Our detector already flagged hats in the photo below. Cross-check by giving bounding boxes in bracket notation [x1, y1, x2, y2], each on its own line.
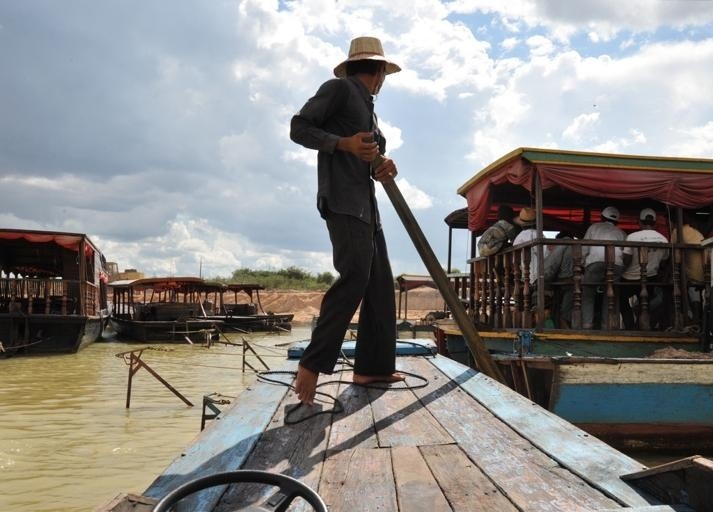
[511, 207, 538, 228]
[637, 207, 657, 224]
[599, 205, 620, 222]
[334, 37, 402, 79]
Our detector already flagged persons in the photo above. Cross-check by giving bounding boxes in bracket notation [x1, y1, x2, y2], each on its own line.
[288, 36, 408, 407]
[477, 204, 713, 331]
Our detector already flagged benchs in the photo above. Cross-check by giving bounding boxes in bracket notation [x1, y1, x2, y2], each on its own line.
[478, 282, 708, 331]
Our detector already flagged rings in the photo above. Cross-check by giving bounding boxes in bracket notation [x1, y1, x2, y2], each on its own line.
[388, 172, 392, 176]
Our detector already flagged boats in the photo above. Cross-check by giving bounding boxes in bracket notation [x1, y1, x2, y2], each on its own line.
[429, 146, 712, 369]
[311, 272, 438, 342]
[0, 229, 295, 355]
[489, 351, 713, 452]
